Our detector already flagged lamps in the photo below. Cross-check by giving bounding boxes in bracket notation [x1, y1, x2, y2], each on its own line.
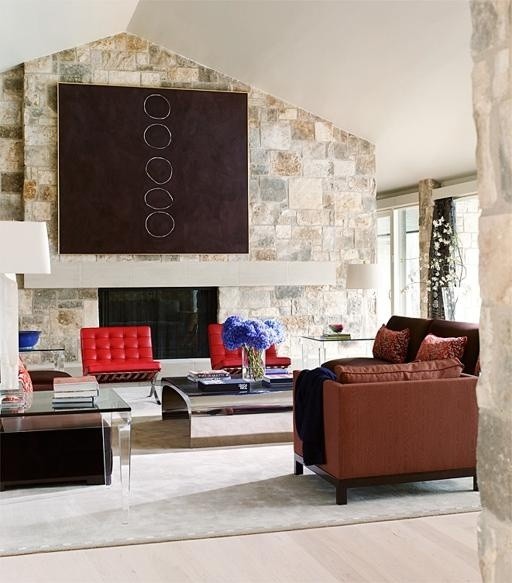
[0, 221, 51, 276]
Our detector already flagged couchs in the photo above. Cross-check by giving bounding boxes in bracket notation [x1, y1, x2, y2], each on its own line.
[291, 316, 480, 506]
[80, 325, 160, 383]
[208, 323, 291, 374]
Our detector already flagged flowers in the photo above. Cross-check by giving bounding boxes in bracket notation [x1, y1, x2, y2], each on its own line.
[222, 315, 286, 351]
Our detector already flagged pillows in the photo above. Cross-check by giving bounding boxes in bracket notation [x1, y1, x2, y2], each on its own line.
[416, 332, 468, 366]
[373, 323, 411, 364]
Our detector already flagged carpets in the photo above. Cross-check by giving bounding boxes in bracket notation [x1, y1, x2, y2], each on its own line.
[1, 381, 480, 557]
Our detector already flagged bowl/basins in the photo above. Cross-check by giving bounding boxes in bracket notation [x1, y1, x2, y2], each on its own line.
[19, 329, 42, 348]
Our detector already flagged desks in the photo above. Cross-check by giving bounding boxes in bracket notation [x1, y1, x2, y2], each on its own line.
[0, 371, 133, 490]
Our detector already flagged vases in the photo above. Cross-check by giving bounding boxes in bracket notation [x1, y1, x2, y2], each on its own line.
[241, 348, 267, 382]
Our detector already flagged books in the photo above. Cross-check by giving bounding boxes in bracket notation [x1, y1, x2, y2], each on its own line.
[186, 369, 231, 383]
[51, 374, 100, 410]
[262, 373, 294, 390]
